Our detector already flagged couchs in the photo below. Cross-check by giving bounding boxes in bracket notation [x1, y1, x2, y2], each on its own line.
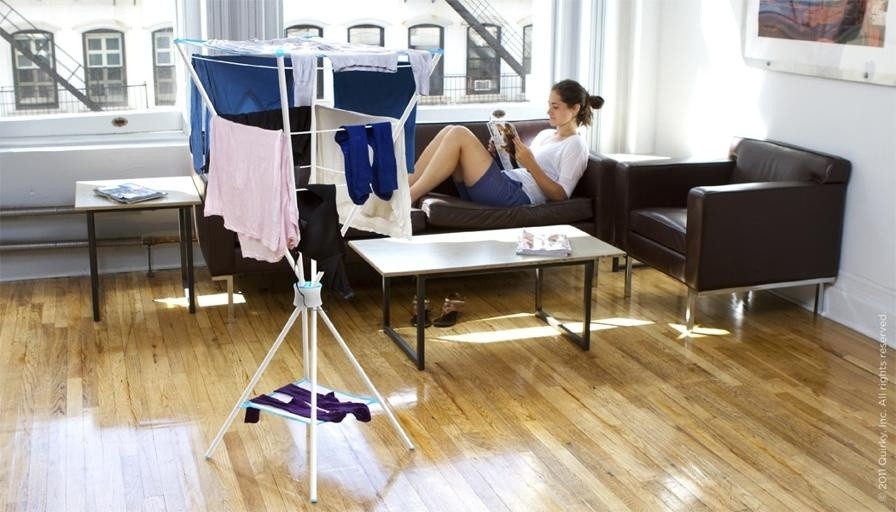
[194, 120, 617, 293]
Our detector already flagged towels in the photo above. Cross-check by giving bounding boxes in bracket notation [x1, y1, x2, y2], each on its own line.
[184, 51, 420, 264]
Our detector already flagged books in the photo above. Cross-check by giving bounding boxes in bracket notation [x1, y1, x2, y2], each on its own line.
[93, 183, 167, 204]
[485, 109, 520, 169]
[517, 229, 572, 258]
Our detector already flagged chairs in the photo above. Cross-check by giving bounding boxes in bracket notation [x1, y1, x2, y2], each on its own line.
[619, 138, 852, 332]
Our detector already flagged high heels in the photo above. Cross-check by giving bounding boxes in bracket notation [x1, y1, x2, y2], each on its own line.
[411, 292, 464, 327]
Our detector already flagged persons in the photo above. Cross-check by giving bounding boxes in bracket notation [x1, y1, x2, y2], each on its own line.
[408, 79, 606, 207]
